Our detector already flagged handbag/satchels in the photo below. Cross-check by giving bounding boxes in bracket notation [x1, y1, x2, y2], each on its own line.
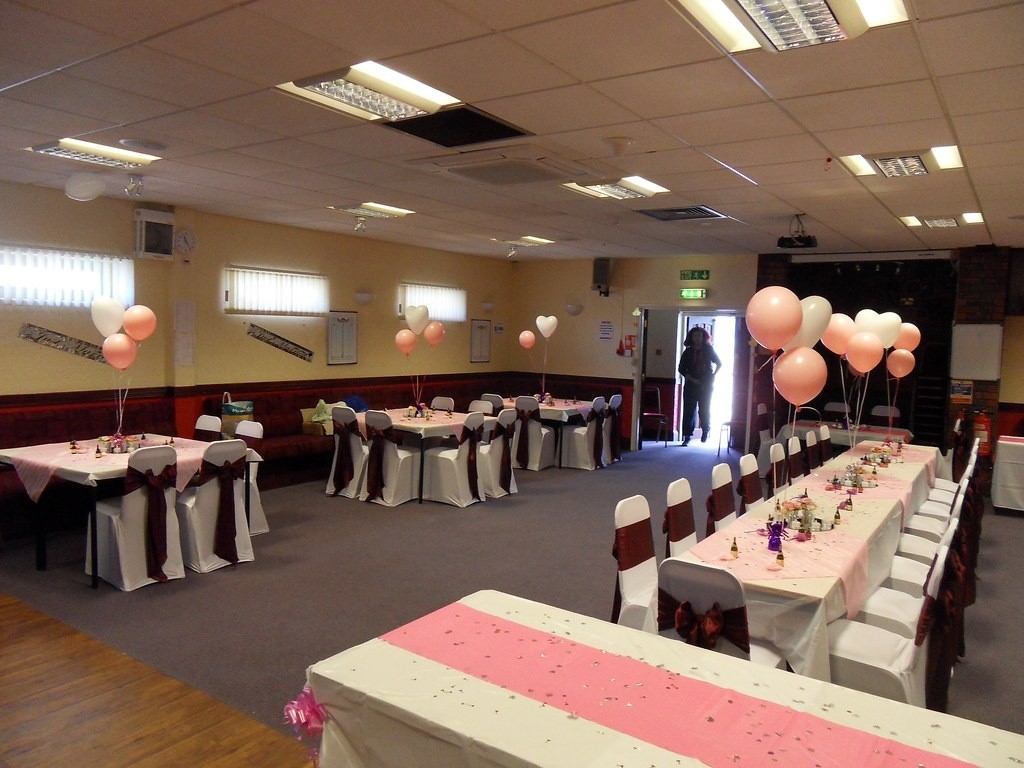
[222, 392, 254, 415]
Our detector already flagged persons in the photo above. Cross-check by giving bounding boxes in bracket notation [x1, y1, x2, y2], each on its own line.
[678, 327, 722, 445]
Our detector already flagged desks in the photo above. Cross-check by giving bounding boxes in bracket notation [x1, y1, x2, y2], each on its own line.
[357, 405, 497, 505]
[501, 396, 606, 466]
[675, 439, 953, 686]
[778, 420, 913, 456]
[306, 588, 1024, 768]
[0, 433, 263, 588]
[992, 436, 1024, 512]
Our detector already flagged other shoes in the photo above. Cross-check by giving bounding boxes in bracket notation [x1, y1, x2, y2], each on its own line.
[682, 436, 690, 446]
[701, 431, 708, 442]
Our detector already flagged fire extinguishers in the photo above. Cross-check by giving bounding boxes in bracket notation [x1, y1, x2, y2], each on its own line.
[973, 408, 992, 456]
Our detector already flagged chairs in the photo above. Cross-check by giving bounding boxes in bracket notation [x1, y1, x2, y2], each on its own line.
[84, 387, 985, 705]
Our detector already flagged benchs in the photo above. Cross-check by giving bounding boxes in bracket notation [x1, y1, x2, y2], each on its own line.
[1, 378, 634, 505]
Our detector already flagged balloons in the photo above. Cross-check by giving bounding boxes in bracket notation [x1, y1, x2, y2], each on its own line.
[102, 333, 138, 372]
[122, 305, 158, 341]
[855, 309, 879, 332]
[405, 305, 428, 336]
[887, 349, 915, 380]
[893, 323, 921, 352]
[536, 316, 558, 338]
[394, 329, 417, 356]
[745, 286, 803, 355]
[879, 312, 902, 349]
[821, 313, 856, 358]
[772, 347, 828, 412]
[519, 330, 536, 349]
[91, 296, 125, 337]
[782, 296, 832, 352]
[423, 321, 447, 349]
[846, 332, 884, 377]
